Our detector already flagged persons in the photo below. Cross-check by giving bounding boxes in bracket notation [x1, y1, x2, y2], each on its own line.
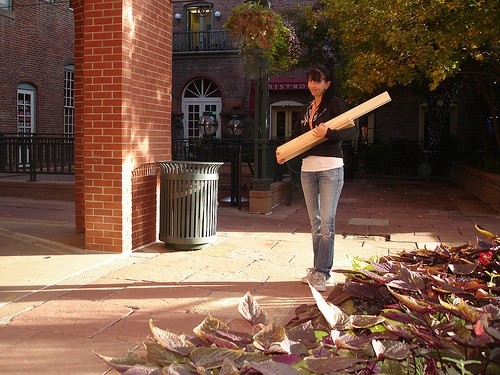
[276, 66, 360, 293]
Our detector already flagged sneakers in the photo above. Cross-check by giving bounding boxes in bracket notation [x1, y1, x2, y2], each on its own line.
[302, 267, 315, 283]
[311, 271, 327, 291]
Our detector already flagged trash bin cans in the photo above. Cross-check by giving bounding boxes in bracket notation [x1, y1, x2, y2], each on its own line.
[156, 161, 224, 250]
